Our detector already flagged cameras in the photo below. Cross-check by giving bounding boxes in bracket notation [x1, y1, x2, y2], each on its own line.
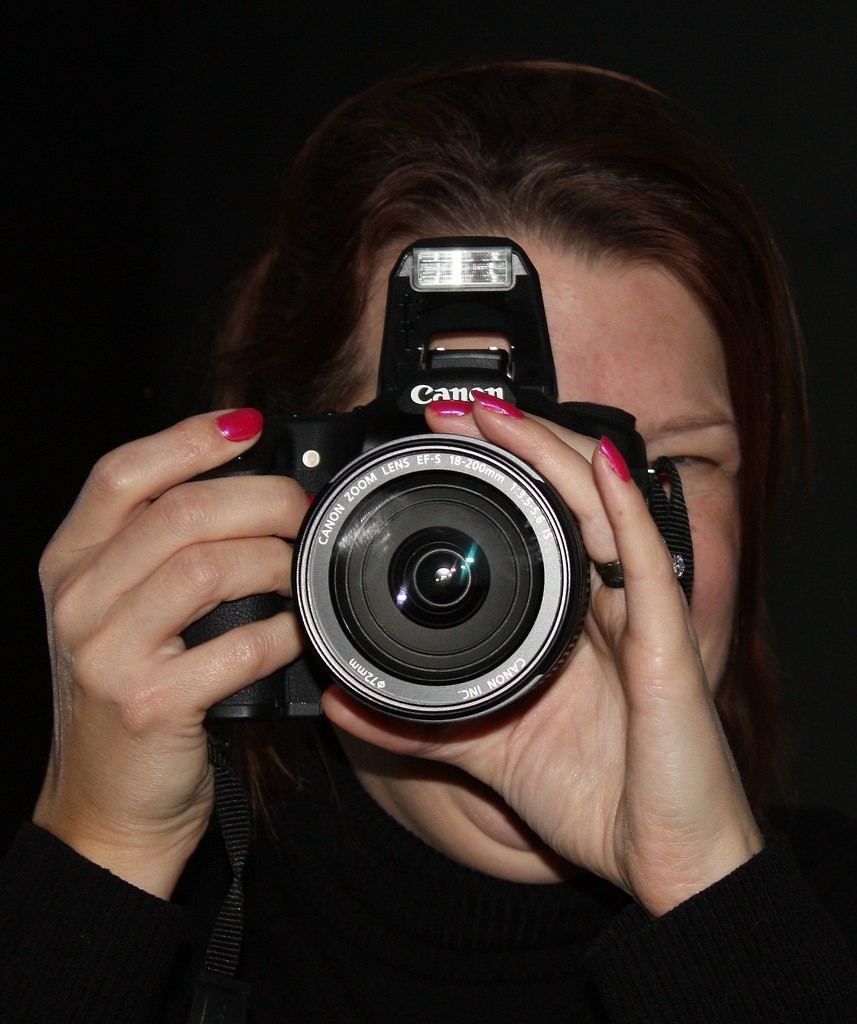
[178, 235, 650, 723]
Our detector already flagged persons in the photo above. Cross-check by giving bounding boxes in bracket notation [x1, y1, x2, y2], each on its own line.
[0, 60, 857, 1024]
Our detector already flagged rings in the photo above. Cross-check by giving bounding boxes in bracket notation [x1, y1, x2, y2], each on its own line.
[594, 554, 686, 588]
